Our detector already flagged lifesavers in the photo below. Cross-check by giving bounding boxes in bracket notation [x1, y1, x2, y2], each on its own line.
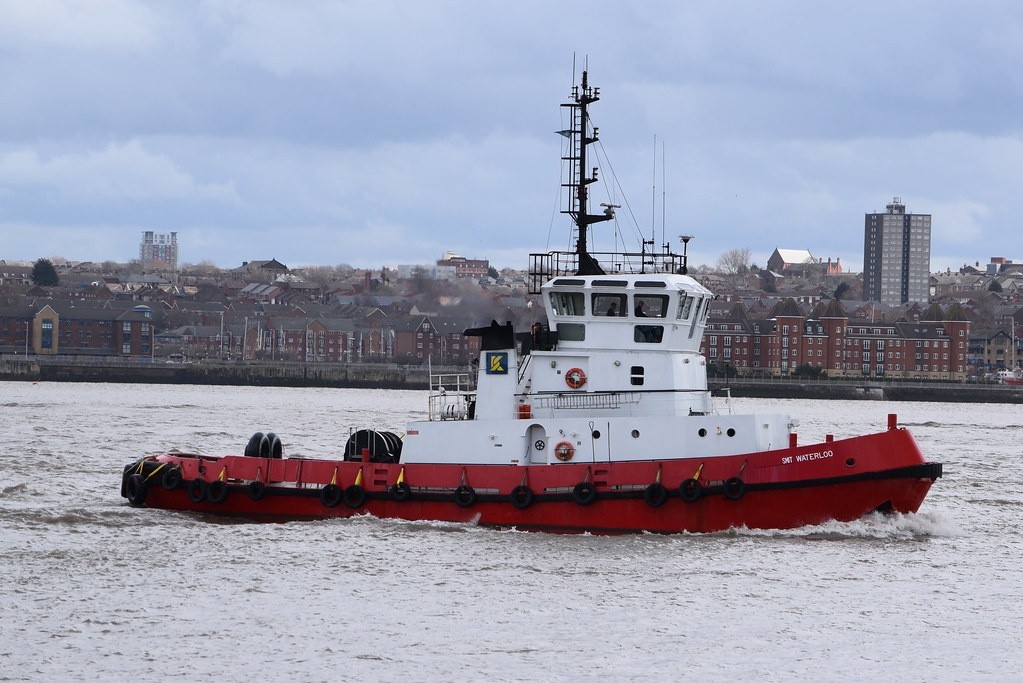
[644, 483, 666, 507]
[162, 469, 182, 491]
[320, 484, 342, 507]
[566, 368, 585, 389]
[206, 481, 227, 502]
[510, 485, 533, 508]
[121, 464, 134, 498]
[679, 478, 703, 502]
[723, 476, 744, 500]
[573, 482, 595, 504]
[125, 474, 146, 506]
[391, 482, 411, 502]
[342, 485, 366, 508]
[247, 481, 265, 500]
[555, 441, 574, 461]
[188, 478, 206, 502]
[454, 485, 475, 507]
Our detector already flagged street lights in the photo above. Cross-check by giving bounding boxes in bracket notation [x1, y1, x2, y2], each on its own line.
[1007, 315, 1016, 370]
[219, 314, 224, 361]
[24, 321, 28, 361]
[243, 315, 247, 362]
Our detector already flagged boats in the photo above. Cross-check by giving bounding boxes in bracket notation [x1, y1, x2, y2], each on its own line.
[118, 49, 944, 524]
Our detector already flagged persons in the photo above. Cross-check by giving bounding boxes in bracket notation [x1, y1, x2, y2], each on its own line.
[607, 303, 617, 316]
[635, 301, 647, 316]
